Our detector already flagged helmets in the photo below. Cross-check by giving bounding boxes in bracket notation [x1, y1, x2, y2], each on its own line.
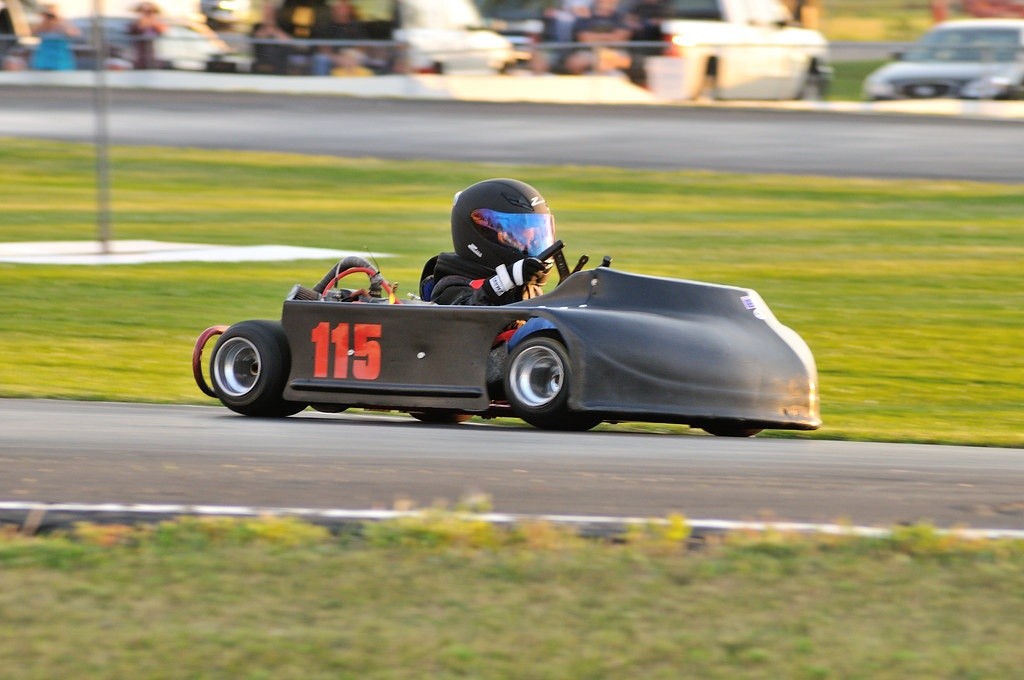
[451, 178, 556, 270]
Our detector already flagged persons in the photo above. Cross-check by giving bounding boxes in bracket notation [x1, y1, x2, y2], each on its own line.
[529, 0, 666, 77]
[0, 0, 405, 83]
[432, 178, 556, 344]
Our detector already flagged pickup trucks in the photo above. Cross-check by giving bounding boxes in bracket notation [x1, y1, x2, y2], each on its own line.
[643, 0, 833, 101]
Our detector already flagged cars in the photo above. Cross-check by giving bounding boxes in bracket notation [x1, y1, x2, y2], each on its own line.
[861, 19, 1024, 101]
[356, 0, 514, 75]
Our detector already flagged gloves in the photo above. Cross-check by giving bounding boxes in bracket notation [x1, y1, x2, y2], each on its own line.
[485, 257, 547, 307]
